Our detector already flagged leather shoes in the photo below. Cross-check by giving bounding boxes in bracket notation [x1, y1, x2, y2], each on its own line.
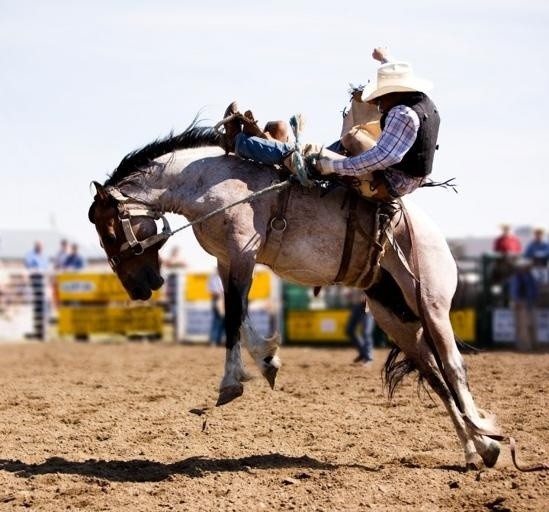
[219, 101, 241, 153]
[243, 110, 263, 137]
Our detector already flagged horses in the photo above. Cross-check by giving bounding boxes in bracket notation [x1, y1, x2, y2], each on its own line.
[86, 114, 502, 474]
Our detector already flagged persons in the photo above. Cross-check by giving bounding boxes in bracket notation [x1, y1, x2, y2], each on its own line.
[220, 44, 442, 204]
[507, 257, 541, 351]
[64, 243, 84, 267]
[495, 225, 523, 256]
[55, 239, 71, 264]
[165, 246, 188, 267]
[24, 240, 53, 268]
[343, 288, 375, 366]
[206, 268, 224, 345]
[525, 228, 549, 285]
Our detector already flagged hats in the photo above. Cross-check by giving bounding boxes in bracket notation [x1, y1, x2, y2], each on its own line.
[361, 62, 433, 103]
